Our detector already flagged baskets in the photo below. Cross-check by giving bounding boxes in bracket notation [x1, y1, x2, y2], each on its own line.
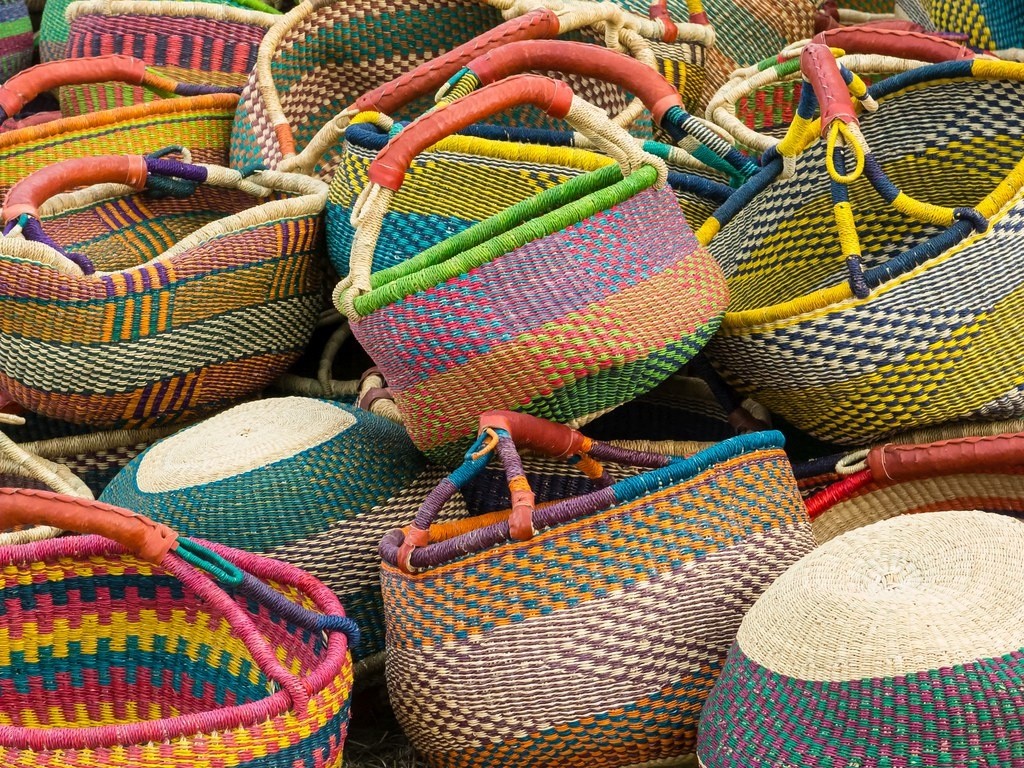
[0, 0, 1024, 768]
